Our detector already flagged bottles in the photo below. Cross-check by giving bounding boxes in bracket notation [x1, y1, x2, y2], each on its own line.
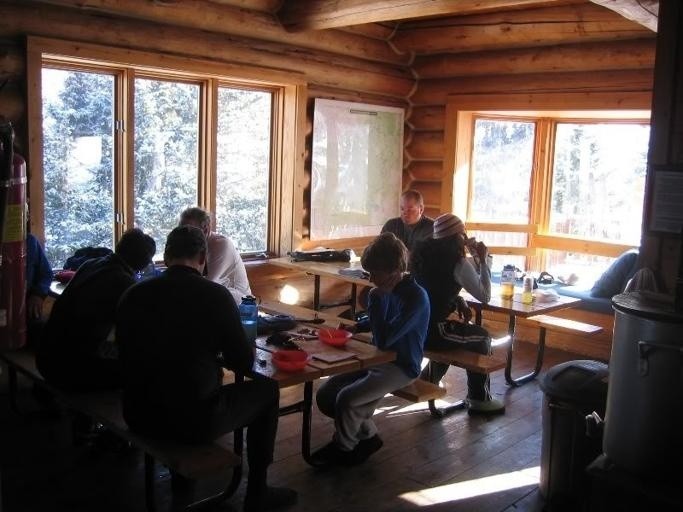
[522, 271, 533, 304]
[500, 263, 515, 300]
[237, 293, 259, 346]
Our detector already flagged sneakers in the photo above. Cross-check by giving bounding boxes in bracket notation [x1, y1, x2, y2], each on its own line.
[310, 433, 384, 472]
[469, 397, 506, 416]
[244, 486, 298, 512]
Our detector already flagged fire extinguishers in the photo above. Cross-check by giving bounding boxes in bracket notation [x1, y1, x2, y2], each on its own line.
[0, 115, 27, 353]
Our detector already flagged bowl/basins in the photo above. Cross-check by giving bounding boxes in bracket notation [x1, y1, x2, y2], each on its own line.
[270, 348, 313, 371]
[315, 327, 352, 346]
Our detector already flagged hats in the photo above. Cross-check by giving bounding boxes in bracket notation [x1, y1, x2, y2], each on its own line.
[433, 213, 465, 240]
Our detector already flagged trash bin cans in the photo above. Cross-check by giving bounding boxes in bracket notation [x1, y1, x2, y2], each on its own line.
[539, 360, 609, 506]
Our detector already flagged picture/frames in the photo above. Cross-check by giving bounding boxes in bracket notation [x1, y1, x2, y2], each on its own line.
[643, 163, 683, 239]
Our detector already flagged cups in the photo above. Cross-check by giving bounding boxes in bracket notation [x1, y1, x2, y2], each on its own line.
[465, 237, 477, 256]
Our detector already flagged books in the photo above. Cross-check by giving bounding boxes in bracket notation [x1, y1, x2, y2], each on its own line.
[337, 264, 369, 279]
[310, 349, 358, 364]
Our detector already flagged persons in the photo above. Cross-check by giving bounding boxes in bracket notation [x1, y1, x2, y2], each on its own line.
[24, 204, 53, 352]
[175, 208, 251, 310]
[412, 213, 505, 412]
[377, 191, 439, 269]
[34, 228, 156, 396]
[119, 223, 298, 510]
[307, 232, 433, 470]
